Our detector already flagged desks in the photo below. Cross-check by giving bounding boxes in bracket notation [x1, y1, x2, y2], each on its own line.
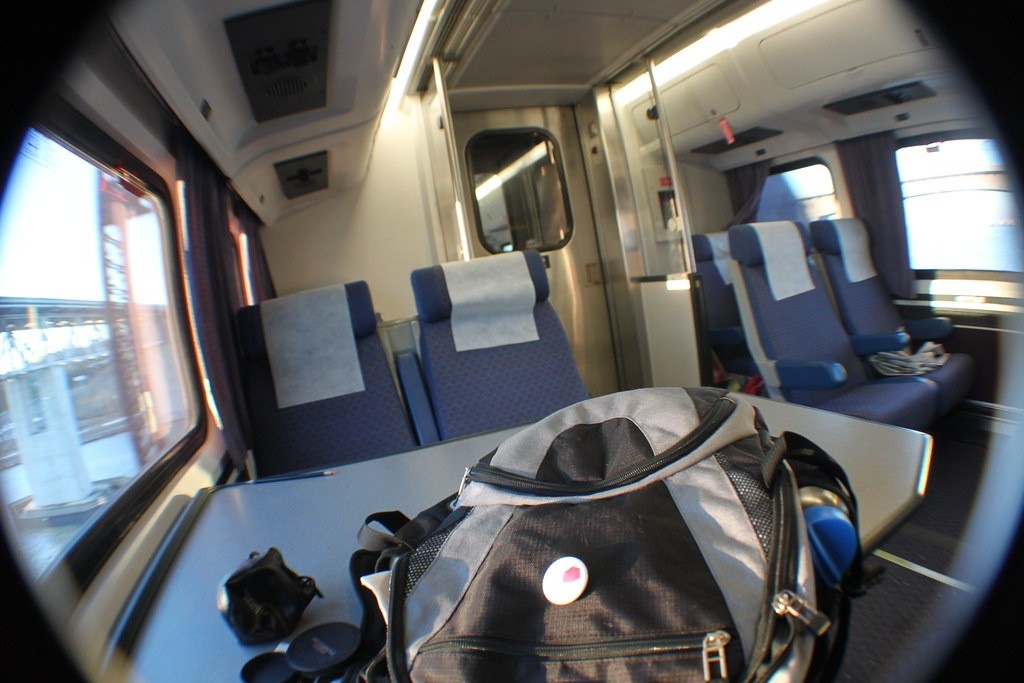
[117, 386, 936, 683]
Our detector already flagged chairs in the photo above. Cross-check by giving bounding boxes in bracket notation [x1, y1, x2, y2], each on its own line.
[409, 250, 591, 442]
[233, 280, 420, 469]
[810, 217, 974, 421]
[691, 230, 759, 379]
[728, 220, 943, 432]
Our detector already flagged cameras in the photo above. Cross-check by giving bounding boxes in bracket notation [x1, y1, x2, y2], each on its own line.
[216, 547, 324, 648]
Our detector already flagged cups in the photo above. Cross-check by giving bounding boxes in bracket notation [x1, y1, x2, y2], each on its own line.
[797, 486, 861, 589]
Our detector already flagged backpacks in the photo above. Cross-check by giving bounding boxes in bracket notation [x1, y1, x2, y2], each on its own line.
[313, 384, 886, 683]
